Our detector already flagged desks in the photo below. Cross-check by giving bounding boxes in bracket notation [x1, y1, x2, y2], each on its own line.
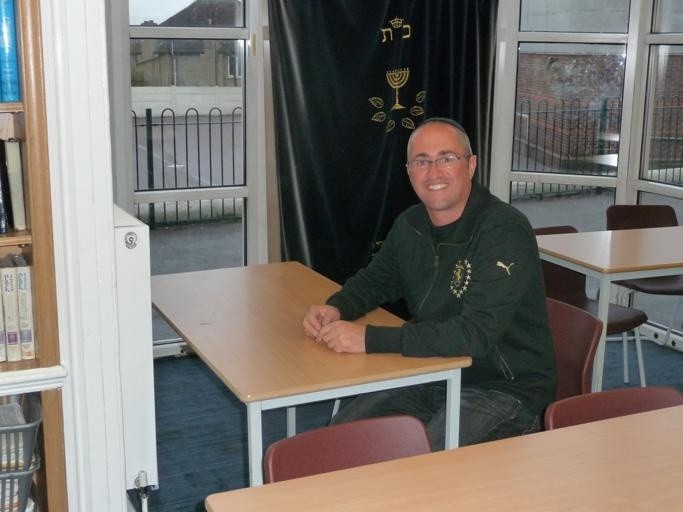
[529, 222, 681, 392]
[148, 260, 474, 490]
[201, 402, 682, 511]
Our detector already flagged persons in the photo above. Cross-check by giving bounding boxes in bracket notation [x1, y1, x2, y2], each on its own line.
[302, 117, 559, 453]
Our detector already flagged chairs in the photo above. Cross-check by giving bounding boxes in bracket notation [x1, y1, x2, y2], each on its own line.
[532, 225, 646, 389]
[543, 297, 603, 399]
[605, 201, 682, 387]
[260, 413, 430, 484]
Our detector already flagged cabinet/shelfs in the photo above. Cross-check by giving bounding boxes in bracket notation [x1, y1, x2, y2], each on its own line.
[0, 1, 72, 512]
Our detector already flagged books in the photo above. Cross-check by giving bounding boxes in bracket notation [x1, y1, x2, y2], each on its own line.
[1, 0, 37, 368]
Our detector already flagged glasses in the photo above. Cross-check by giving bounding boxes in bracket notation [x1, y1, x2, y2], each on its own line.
[405, 153, 471, 170]
